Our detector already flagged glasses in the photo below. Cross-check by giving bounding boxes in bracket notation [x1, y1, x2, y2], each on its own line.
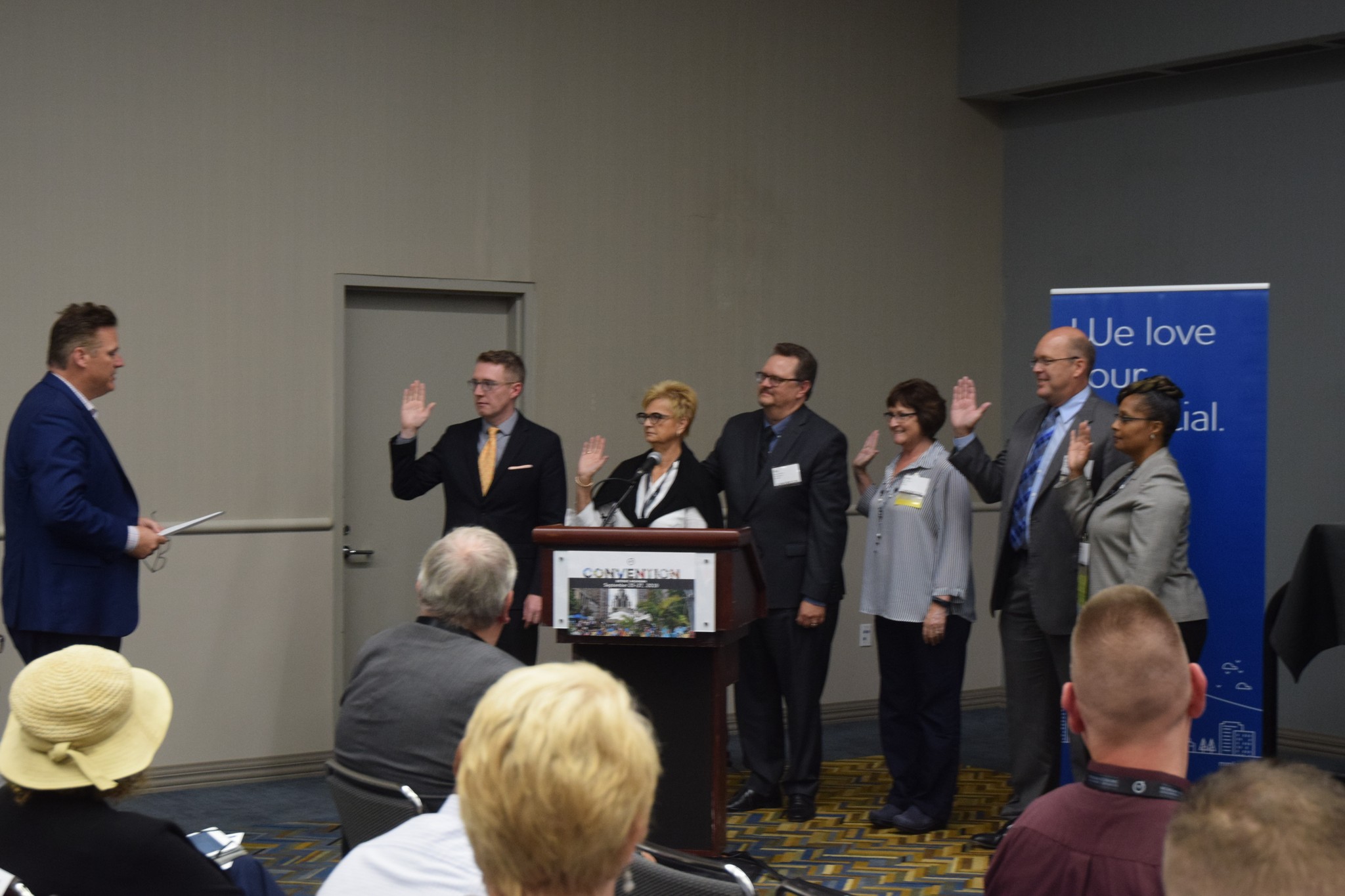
[466, 379, 513, 391]
[1113, 412, 1143, 423]
[755, 371, 800, 385]
[1029, 355, 1078, 365]
[883, 410, 913, 421]
[635, 412, 668, 424]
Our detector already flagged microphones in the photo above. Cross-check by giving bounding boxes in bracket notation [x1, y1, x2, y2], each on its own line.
[633, 452, 662, 481]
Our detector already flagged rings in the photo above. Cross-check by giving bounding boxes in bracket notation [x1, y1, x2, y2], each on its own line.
[587, 451, 592, 454]
[934, 632, 941, 634]
[810, 624, 818, 626]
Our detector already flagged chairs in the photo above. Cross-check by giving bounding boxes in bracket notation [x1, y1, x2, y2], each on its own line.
[615, 841, 857, 896]
[324, 759, 426, 862]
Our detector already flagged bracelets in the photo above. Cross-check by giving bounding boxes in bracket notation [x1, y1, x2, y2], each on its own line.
[575, 477, 594, 486]
[932, 597, 951, 607]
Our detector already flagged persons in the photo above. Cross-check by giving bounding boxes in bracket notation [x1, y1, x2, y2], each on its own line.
[1052, 376, 1211, 763]
[852, 378, 977, 833]
[333, 527, 527, 812]
[390, 352, 567, 667]
[312, 740, 487, 896]
[947, 325, 1132, 848]
[454, 662, 658, 896]
[1161, 759, 1345, 896]
[0, 645, 286, 896]
[700, 343, 851, 822]
[983, 583, 1207, 896]
[1, 302, 166, 665]
[573, 379, 723, 529]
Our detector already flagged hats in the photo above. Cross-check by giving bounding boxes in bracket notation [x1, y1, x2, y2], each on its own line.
[1, 644, 172, 791]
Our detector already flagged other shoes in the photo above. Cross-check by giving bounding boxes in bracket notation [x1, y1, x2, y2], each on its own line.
[868, 803, 901, 825]
[969, 820, 1016, 849]
[894, 806, 944, 832]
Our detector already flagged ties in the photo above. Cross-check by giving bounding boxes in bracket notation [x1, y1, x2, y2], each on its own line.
[759, 427, 771, 472]
[1009, 410, 1060, 551]
[478, 427, 499, 496]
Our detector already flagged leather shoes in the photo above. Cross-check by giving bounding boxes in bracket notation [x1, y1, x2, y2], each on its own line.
[726, 789, 782, 810]
[787, 793, 816, 821]
